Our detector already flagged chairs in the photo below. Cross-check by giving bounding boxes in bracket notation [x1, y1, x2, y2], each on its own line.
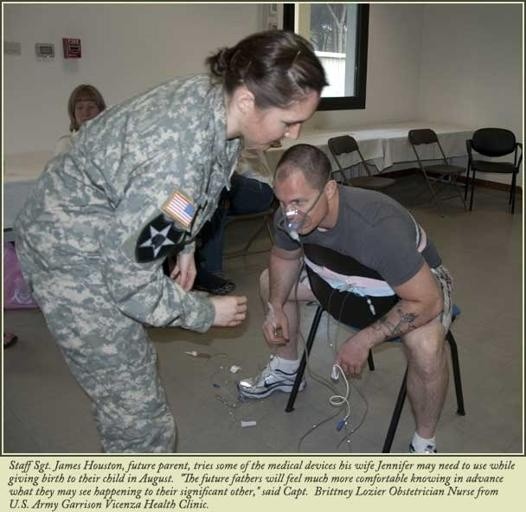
[408, 127, 522, 219]
[282, 243, 466, 454]
[327, 134, 397, 193]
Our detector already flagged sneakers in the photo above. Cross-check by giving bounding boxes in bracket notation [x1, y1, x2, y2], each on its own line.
[192, 276, 236, 296]
[236, 365, 304, 399]
[409, 442, 437, 453]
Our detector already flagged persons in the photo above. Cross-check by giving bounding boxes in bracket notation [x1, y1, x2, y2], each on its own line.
[234, 143, 454, 453]
[4, 331, 18, 348]
[66, 82, 239, 297]
[196, 139, 276, 277]
[14, 29, 331, 454]
